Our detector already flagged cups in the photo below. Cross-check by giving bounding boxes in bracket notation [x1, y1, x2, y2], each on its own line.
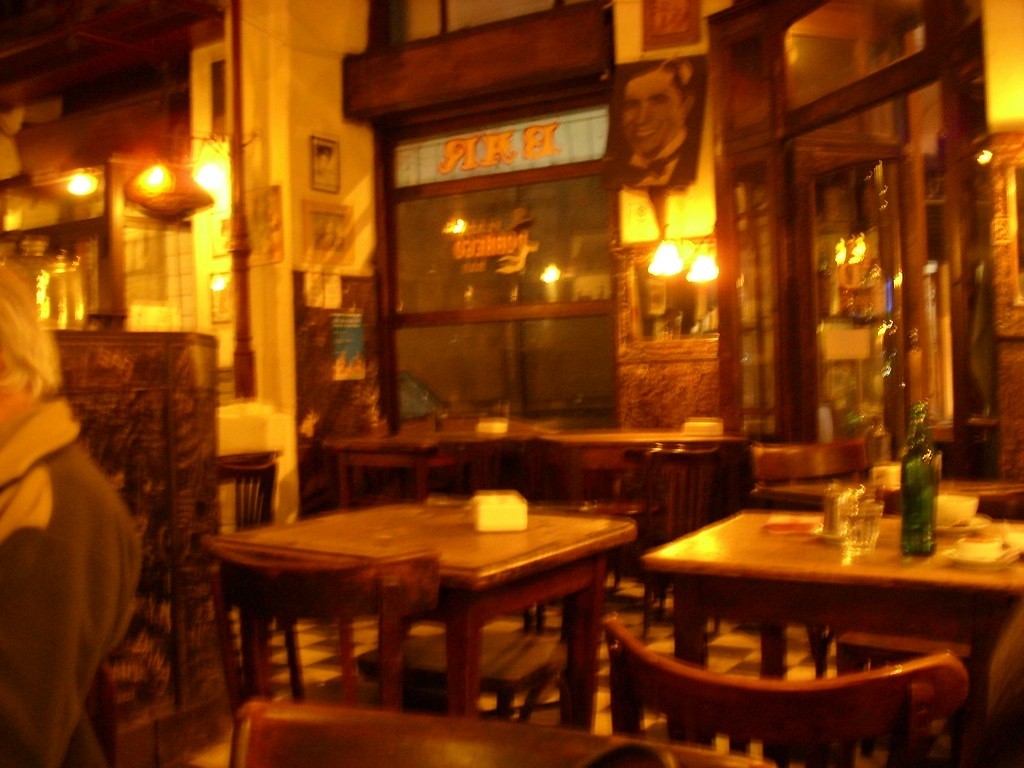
[936, 494, 979, 527]
[957, 533, 1003, 559]
[871, 463, 901, 492]
[820, 479, 884, 556]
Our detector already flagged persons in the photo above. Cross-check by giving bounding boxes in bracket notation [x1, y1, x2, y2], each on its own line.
[613, 59, 697, 184]
[0, 267, 139, 768]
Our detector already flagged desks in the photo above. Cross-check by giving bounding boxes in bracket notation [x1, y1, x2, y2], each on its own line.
[321, 435, 529, 515]
[642, 508, 1024, 767]
[529, 429, 749, 527]
[750, 470, 1024, 519]
[211, 497, 634, 733]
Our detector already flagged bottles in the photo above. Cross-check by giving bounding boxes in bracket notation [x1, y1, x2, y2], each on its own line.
[901, 398, 938, 554]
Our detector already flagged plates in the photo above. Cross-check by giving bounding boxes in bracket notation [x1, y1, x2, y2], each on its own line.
[943, 544, 1022, 571]
[935, 519, 990, 535]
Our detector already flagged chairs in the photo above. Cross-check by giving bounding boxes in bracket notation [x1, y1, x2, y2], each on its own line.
[202, 412, 1024, 768]
